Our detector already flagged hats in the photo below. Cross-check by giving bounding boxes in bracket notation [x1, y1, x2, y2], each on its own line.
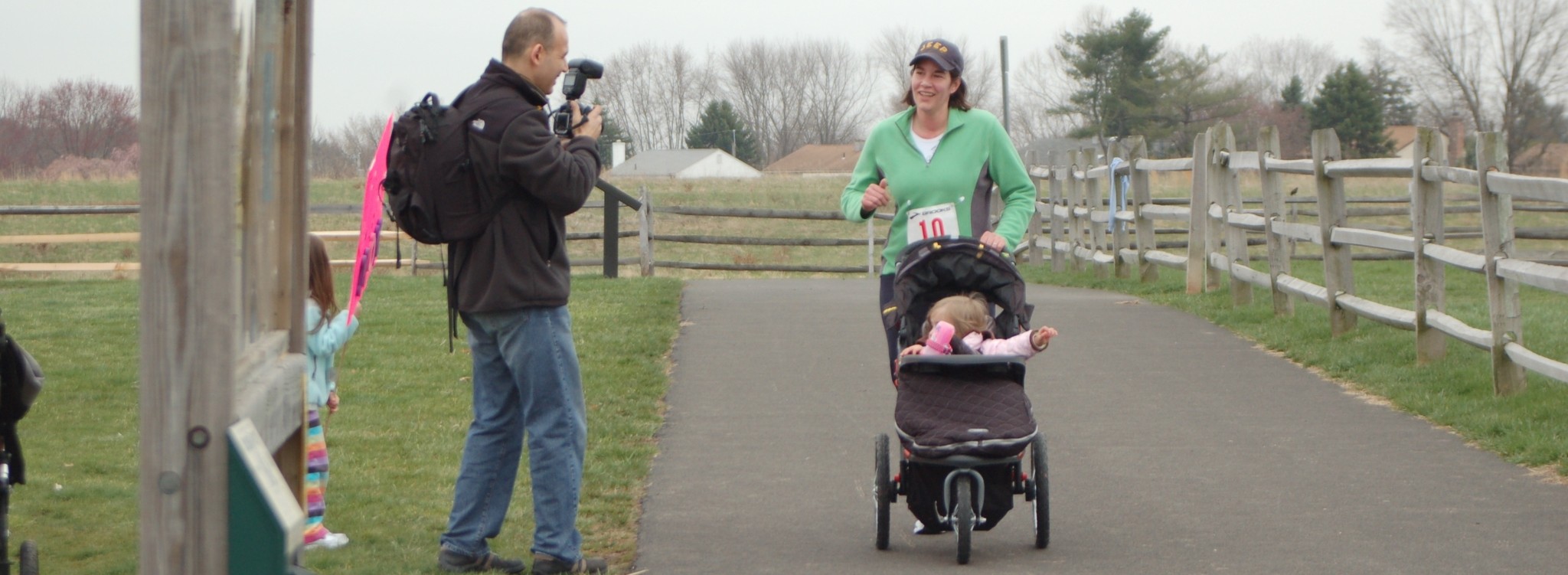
[910, 38, 965, 76]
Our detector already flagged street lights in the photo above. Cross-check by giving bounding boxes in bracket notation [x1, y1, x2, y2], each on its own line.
[732, 129, 736, 158]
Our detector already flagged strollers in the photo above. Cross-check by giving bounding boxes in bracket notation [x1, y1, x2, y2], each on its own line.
[871, 234, 1052, 565]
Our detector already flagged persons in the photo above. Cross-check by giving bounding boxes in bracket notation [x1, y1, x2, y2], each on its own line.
[302, 234, 361, 550]
[898, 295, 1057, 360]
[436, 7, 608, 575]
[839, 39, 1037, 536]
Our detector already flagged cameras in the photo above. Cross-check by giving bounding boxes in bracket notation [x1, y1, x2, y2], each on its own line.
[554, 57, 605, 139]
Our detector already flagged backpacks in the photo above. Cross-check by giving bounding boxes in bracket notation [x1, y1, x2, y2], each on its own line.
[388, 87, 521, 247]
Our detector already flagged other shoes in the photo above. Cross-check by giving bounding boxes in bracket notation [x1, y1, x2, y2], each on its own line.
[914, 519, 943, 534]
[438, 546, 527, 573]
[306, 534, 348, 550]
[532, 550, 606, 575]
